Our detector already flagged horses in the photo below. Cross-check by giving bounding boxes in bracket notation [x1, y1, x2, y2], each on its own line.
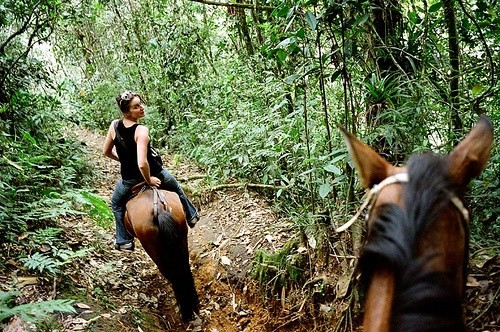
[339, 113, 495, 332]
[124, 183, 200, 325]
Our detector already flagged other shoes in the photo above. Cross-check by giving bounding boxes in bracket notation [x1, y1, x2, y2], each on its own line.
[114, 242, 135, 250]
[188, 212, 200, 227]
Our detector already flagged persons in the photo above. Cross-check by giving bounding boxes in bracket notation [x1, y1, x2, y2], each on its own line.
[103, 91, 200, 252]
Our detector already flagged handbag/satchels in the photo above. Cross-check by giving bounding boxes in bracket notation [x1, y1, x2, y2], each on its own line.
[147, 147, 163, 173]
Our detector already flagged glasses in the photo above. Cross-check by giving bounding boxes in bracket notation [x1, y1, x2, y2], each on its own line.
[119, 89, 134, 107]
